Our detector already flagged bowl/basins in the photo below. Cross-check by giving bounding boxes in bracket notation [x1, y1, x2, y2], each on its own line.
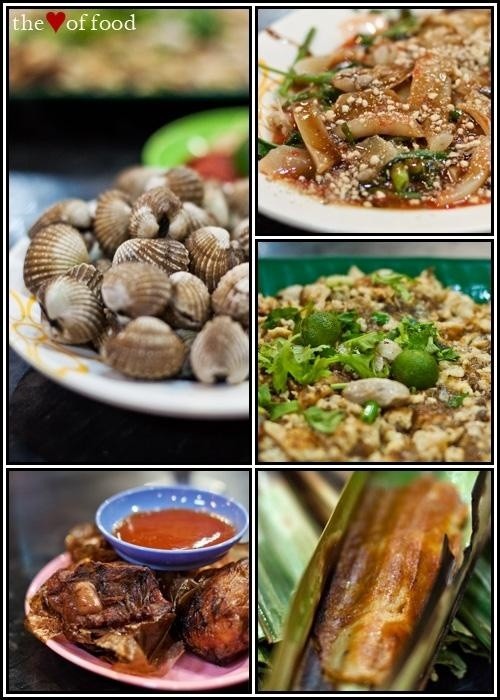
[94, 485, 247, 567]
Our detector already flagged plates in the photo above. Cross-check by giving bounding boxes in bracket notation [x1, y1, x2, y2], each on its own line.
[142, 109, 251, 183]
[8, 219, 252, 424]
[25, 541, 252, 689]
[257, 6, 493, 234]
